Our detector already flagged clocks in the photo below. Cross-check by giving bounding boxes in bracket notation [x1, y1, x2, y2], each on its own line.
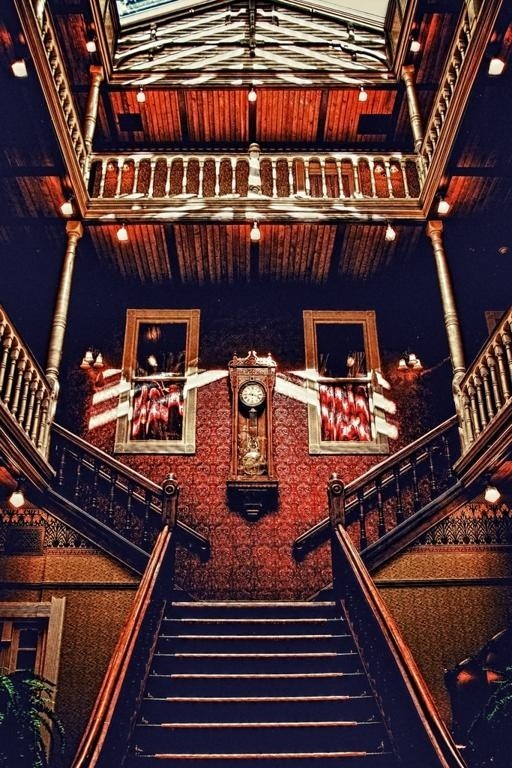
[226, 347, 280, 520]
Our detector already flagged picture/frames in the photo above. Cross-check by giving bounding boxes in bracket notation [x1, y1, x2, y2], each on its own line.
[302, 309, 390, 457]
[111, 308, 201, 458]
